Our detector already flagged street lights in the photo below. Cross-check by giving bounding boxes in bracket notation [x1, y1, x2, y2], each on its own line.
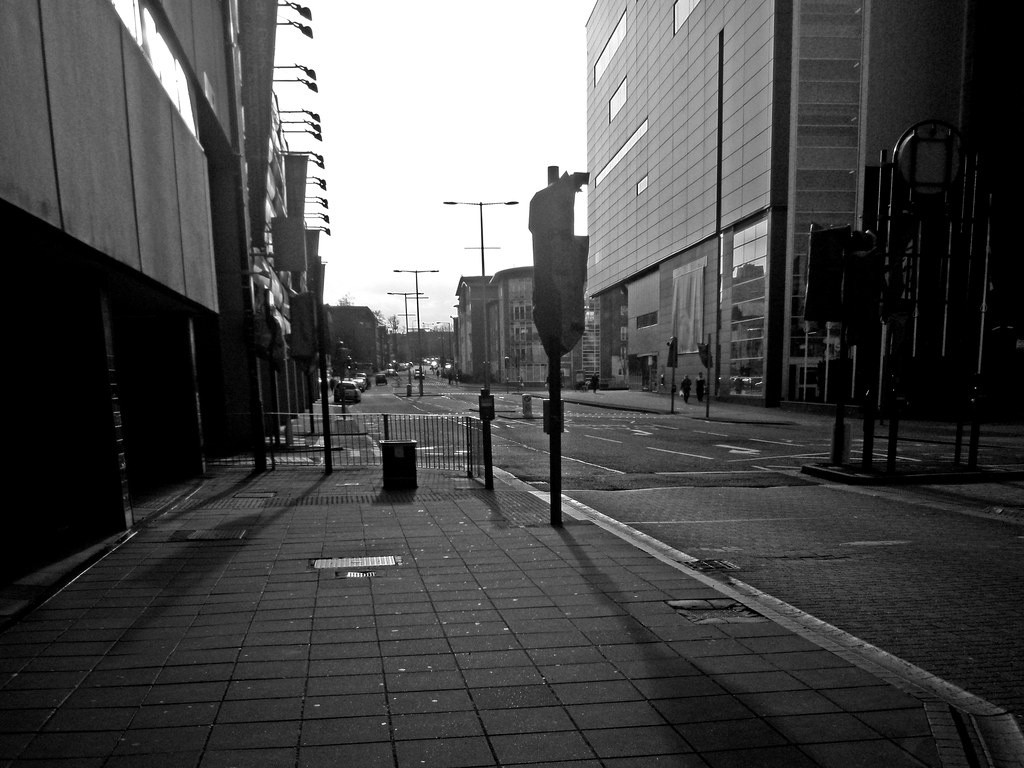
[394, 270, 439, 396]
[443, 201, 519, 396]
[387, 293, 423, 385]
[436, 320, 452, 357]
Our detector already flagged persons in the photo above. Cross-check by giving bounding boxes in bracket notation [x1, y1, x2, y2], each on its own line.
[695, 372, 705, 402]
[592, 373, 598, 393]
[681, 375, 691, 403]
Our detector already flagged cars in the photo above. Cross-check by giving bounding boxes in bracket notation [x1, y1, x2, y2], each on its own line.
[388, 359, 414, 376]
[343, 371, 369, 393]
[413, 369, 425, 380]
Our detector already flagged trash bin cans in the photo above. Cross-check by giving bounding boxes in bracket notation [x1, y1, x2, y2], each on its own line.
[380, 439, 418, 491]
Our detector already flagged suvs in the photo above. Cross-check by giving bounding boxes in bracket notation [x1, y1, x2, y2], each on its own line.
[375, 374, 388, 385]
[333, 380, 363, 403]
